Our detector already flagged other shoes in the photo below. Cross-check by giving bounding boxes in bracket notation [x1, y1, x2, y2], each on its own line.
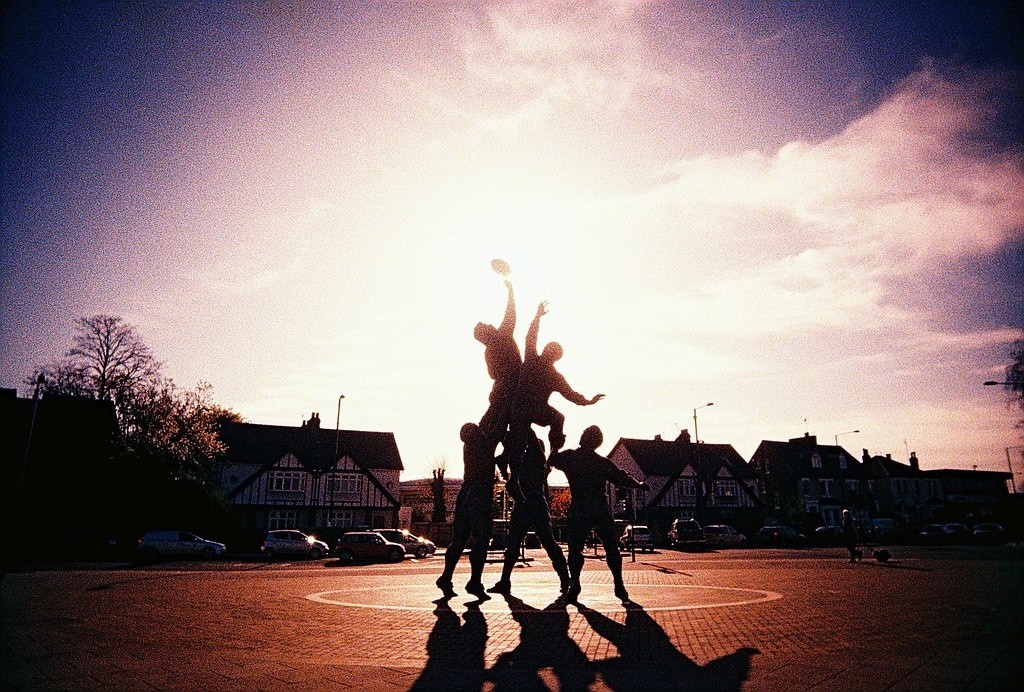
[436, 576, 458, 596]
[505, 479, 526, 502]
[615, 587, 629, 600]
[496, 455, 509, 480]
[465, 581, 490, 599]
[564, 585, 581, 601]
[487, 582, 510, 592]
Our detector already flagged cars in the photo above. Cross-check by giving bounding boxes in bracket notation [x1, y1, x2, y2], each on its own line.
[919, 523, 1007, 546]
[756, 518, 904, 548]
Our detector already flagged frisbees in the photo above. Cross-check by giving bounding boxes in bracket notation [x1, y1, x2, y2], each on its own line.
[491, 257, 511, 275]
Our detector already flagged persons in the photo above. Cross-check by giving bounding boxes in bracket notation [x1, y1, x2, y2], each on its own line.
[839, 509, 863, 563]
[436, 281, 650, 600]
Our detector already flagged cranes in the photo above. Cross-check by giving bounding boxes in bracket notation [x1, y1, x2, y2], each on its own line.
[619, 524, 653, 551]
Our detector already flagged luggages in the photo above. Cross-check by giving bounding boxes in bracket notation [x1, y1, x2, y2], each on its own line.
[864, 544, 891, 563]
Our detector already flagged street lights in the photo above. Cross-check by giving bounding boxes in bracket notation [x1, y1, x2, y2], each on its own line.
[836, 430, 859, 446]
[329, 394, 346, 525]
[694, 403, 713, 442]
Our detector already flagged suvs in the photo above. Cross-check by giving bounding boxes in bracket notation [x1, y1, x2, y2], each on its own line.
[334, 529, 437, 562]
[134, 528, 227, 564]
[525, 532, 541, 549]
[261, 530, 330, 559]
[668, 518, 748, 549]
